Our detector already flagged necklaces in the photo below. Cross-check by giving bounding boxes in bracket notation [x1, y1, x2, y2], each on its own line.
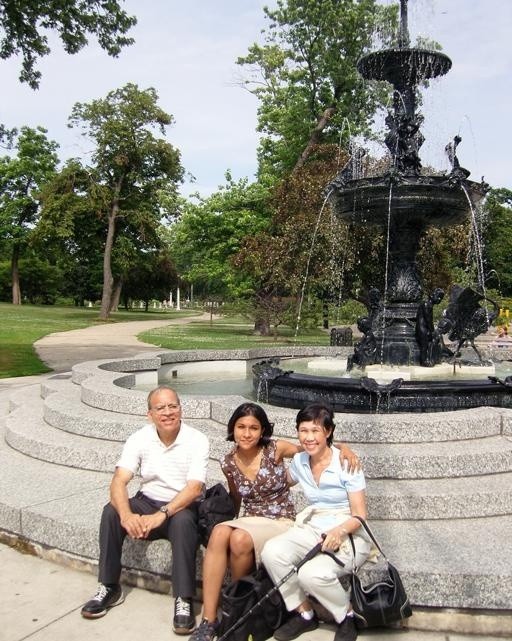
[237, 447, 258, 476]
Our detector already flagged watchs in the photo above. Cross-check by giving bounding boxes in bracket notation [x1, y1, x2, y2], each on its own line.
[160, 506, 169, 520]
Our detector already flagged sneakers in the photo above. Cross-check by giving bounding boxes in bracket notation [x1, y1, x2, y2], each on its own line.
[188, 615, 220, 641]
[172, 597, 198, 635]
[80, 581, 124, 619]
[334, 613, 361, 641]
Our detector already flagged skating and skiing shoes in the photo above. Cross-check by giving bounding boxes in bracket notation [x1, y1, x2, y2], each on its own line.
[273, 610, 321, 640]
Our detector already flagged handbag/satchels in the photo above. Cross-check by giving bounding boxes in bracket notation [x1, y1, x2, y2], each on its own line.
[218, 562, 283, 641]
[347, 515, 414, 622]
[197, 483, 237, 547]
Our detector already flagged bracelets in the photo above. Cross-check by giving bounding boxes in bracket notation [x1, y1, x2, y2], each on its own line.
[338, 525, 346, 535]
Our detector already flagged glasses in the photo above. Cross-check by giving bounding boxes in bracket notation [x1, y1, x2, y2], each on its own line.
[155, 403, 180, 415]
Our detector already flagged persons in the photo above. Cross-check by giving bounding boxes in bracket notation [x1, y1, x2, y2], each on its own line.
[346, 288, 382, 373]
[444, 135, 462, 167]
[262, 404, 369, 640]
[189, 402, 363, 640]
[495, 326, 512, 339]
[416, 287, 454, 366]
[81, 386, 210, 634]
[310, 299, 329, 329]
